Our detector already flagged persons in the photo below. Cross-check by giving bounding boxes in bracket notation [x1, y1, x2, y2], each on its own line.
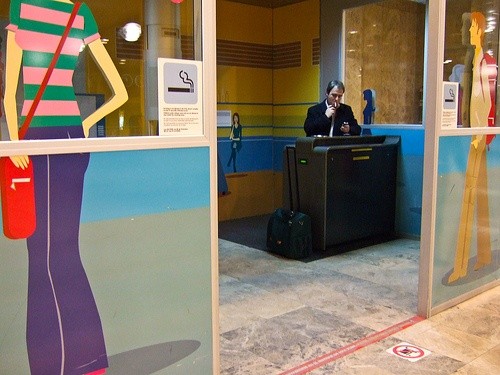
[227, 113, 244, 171]
[361, 89, 377, 137]
[304, 80, 362, 137]
[3, 2, 132, 375]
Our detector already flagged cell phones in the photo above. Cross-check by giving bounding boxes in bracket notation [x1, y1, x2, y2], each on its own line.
[342, 121, 348, 127]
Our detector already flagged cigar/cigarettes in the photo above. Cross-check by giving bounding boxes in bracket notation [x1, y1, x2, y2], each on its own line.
[334, 101, 337, 107]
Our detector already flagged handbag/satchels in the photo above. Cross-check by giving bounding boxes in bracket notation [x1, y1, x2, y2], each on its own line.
[268, 208, 312, 258]
[486, 106, 496, 143]
[1, 156, 37, 240]
